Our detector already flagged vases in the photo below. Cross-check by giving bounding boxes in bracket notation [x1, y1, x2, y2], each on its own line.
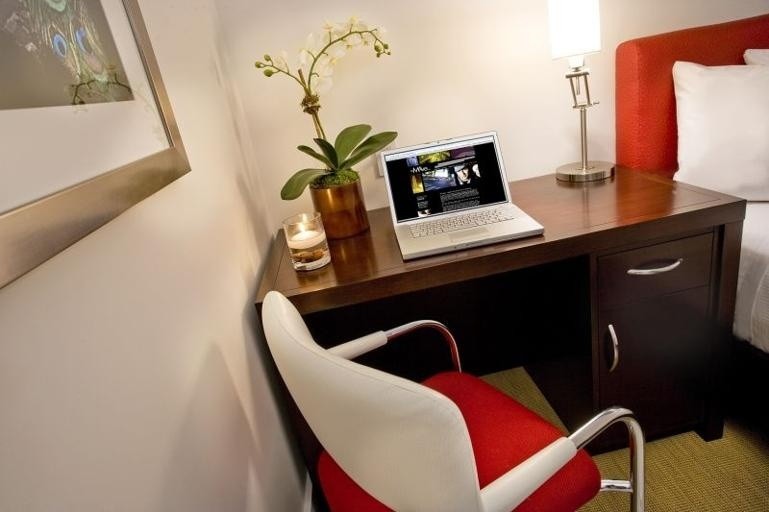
[307, 176, 371, 241]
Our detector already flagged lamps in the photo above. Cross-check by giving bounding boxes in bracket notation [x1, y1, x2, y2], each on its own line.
[545, 0, 617, 185]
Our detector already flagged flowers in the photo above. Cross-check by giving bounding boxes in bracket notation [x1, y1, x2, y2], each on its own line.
[253, 16, 398, 201]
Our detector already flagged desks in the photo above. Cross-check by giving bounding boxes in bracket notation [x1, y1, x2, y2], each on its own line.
[253, 169, 746, 446]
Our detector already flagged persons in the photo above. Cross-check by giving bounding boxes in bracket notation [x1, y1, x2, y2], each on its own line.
[454, 162, 481, 185]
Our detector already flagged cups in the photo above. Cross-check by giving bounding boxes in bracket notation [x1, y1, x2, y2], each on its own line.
[280, 211, 332, 273]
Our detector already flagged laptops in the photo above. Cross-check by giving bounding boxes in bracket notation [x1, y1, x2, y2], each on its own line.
[380, 130, 545, 261]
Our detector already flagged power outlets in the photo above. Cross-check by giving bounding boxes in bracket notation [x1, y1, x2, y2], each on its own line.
[377, 138, 400, 178]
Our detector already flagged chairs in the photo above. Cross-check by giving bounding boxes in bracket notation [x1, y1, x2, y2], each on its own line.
[260, 290, 647, 512]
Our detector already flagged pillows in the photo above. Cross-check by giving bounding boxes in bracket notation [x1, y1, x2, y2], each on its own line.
[672, 47, 769, 202]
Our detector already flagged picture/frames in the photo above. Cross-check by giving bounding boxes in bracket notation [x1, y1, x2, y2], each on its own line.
[0, 0, 194, 293]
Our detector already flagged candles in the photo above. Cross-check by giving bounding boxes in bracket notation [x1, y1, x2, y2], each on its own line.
[283, 221, 326, 249]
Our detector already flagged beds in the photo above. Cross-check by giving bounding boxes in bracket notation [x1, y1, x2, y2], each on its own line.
[614, 11, 769, 361]
